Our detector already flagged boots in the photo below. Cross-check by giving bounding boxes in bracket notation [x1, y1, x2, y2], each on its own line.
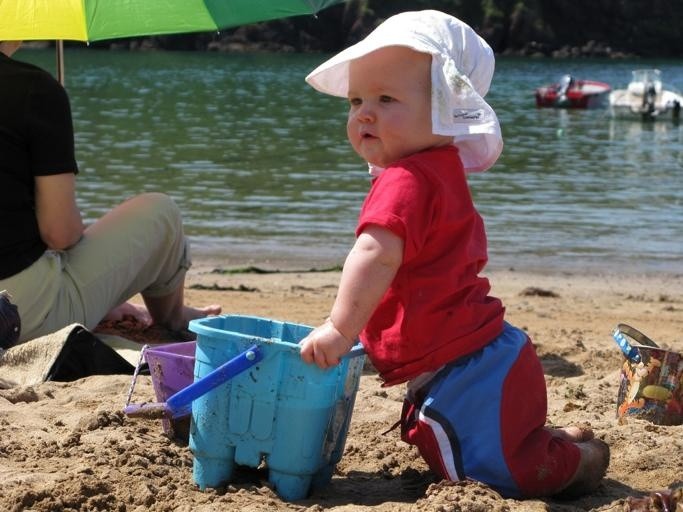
[551, 427, 610, 495]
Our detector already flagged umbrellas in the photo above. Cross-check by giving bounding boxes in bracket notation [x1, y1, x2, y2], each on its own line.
[0, 2, 338, 88]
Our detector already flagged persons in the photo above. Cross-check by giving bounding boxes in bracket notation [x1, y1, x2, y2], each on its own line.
[298, 10, 610, 501]
[0, 40, 222, 351]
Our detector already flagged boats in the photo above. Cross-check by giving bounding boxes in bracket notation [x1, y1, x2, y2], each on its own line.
[534, 69, 683, 123]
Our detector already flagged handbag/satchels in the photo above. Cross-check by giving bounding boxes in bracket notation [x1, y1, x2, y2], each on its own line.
[0, 296, 21, 349]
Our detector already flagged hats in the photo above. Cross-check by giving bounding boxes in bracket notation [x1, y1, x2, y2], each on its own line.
[304, 10, 504, 177]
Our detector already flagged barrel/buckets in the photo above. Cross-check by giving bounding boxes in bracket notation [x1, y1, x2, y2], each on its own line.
[612, 323, 683, 425]
[165, 313, 367, 500]
